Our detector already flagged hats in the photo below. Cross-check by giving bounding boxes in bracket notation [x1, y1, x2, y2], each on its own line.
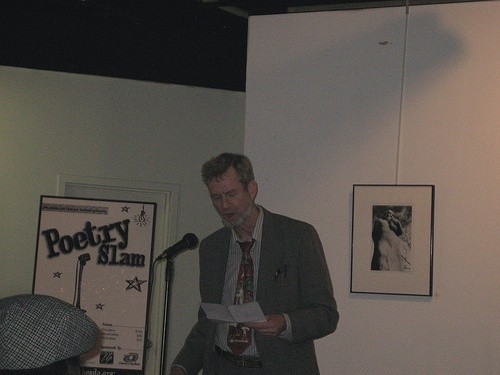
[1, 294, 101, 370]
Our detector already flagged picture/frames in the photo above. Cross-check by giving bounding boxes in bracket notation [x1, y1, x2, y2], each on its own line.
[350, 183, 436, 299]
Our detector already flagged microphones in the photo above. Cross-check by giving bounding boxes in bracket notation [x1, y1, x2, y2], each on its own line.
[78, 252, 90, 262]
[156, 233, 198, 260]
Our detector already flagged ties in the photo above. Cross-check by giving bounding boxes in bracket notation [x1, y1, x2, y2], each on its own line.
[226, 238, 257, 355]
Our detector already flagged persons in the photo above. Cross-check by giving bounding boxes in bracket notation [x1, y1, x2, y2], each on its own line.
[372, 210, 403, 270]
[168, 152, 339, 375]
